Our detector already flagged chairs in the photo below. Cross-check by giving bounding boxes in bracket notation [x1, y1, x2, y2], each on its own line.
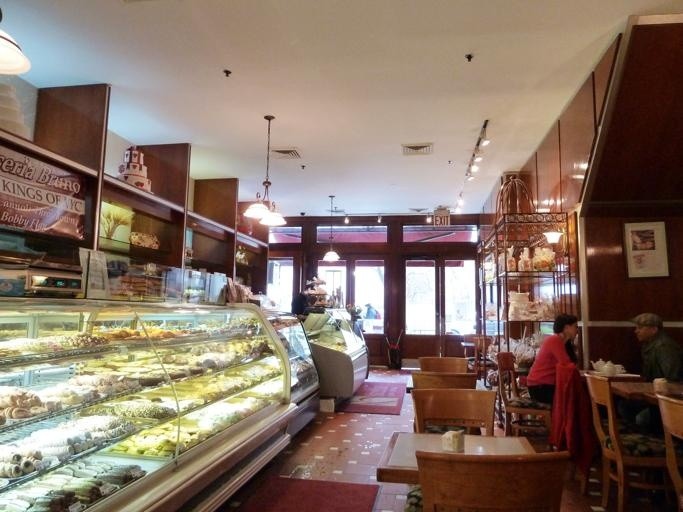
[496, 350, 559, 437]
[411, 387, 495, 435]
[653, 394, 683, 511]
[417, 357, 467, 375]
[412, 371, 477, 391]
[416, 450, 572, 511]
[583, 371, 683, 510]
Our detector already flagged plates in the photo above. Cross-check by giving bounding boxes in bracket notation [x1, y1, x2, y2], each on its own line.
[616, 370, 627, 374]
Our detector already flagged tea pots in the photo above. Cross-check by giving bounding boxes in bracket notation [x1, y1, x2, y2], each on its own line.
[591, 358, 605, 372]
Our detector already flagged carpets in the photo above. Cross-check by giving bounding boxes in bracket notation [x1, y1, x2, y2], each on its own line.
[338, 382, 408, 416]
[248, 468, 379, 512]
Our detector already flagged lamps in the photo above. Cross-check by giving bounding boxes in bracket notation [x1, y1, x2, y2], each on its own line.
[0, 7, 31, 76]
[258, 206, 286, 226]
[426, 119, 491, 223]
[242, 111, 275, 216]
[543, 231, 563, 244]
[323, 195, 341, 262]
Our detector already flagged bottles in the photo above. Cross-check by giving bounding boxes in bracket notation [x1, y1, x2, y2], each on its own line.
[506, 247, 555, 271]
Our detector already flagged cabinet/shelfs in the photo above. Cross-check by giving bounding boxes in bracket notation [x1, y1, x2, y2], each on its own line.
[477, 213, 573, 405]
[1, 296, 321, 512]
[1, 87, 270, 303]
[302, 276, 329, 308]
[302, 308, 371, 399]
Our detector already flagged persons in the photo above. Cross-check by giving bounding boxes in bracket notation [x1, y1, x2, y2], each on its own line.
[629, 312, 683, 512]
[292, 286, 314, 314]
[364, 304, 381, 319]
[526, 313, 578, 405]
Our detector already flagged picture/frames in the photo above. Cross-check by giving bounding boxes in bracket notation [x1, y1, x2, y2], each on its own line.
[624, 222, 670, 278]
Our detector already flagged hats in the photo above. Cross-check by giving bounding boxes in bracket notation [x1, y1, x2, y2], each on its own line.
[628, 312, 663, 328]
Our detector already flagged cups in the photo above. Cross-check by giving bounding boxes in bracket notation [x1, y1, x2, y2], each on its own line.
[654, 379, 668, 393]
[614, 365, 625, 372]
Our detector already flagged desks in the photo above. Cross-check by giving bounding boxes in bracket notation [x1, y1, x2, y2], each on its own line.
[376, 431, 540, 483]
